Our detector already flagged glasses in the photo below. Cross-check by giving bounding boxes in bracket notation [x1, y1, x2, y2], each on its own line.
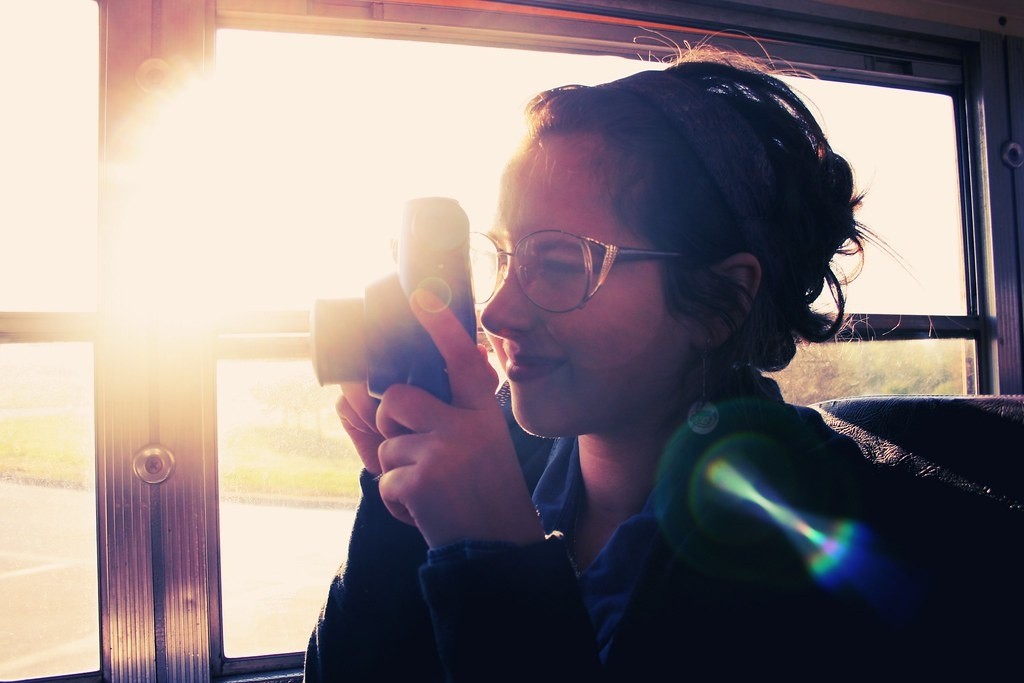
[465, 229, 684, 314]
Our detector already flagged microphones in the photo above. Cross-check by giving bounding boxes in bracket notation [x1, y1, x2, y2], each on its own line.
[652, 402, 843, 589]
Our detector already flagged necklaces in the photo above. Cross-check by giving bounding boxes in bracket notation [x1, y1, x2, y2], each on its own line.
[572, 493, 582, 572]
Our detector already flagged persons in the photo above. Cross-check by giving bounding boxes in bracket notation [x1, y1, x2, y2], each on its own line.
[304, 44, 945, 683]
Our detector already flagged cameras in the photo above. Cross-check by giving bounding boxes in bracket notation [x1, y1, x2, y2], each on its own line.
[307, 198, 476, 407]
